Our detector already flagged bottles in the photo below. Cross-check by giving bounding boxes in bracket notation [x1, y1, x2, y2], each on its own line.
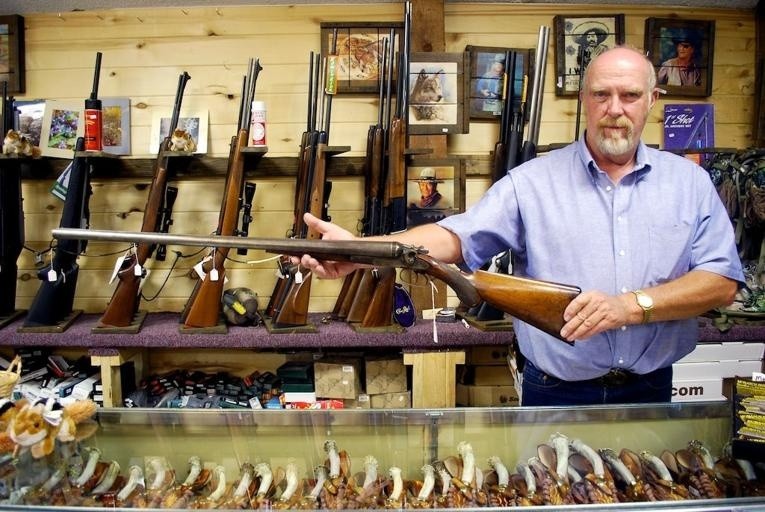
[84, 99, 103, 152]
[251, 101, 268, 147]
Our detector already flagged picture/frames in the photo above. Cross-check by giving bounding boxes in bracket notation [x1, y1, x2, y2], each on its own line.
[1, 14, 25, 94]
[553, 13, 625, 97]
[320, 21, 406, 93]
[644, 17, 715, 97]
[151, 108, 209, 155]
[397, 51, 469, 134]
[467, 45, 535, 121]
[40, 99, 85, 160]
[100, 97, 132, 155]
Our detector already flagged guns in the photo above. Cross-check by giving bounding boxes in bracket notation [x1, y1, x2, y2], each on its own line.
[22, 60, 103, 326]
[331, 1, 413, 326]
[51, 227, 581, 347]
[263, 28, 338, 325]
[0, 80, 24, 314]
[98, 71, 191, 328]
[180, 58, 263, 330]
[456, 27, 549, 318]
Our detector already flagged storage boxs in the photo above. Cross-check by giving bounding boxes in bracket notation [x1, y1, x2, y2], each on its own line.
[365, 359, 408, 394]
[314, 362, 362, 399]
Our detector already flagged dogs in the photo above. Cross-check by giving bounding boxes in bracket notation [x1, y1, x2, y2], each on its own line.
[409, 69, 445, 121]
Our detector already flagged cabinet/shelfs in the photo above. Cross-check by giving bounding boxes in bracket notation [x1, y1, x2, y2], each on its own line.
[1, 401, 764, 512]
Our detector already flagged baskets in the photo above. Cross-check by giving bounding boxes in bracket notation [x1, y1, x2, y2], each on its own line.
[0, 355, 22, 397]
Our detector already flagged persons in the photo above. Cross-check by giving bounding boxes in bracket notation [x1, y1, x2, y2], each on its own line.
[408, 168, 450, 207]
[300, 48, 745, 405]
[657, 34, 701, 86]
[477, 54, 506, 111]
[570, 21, 609, 68]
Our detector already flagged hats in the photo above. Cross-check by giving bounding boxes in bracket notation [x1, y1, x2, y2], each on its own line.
[572, 21, 609, 45]
[671, 32, 697, 44]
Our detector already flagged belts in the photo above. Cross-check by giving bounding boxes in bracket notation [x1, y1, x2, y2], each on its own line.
[598, 365, 636, 384]
